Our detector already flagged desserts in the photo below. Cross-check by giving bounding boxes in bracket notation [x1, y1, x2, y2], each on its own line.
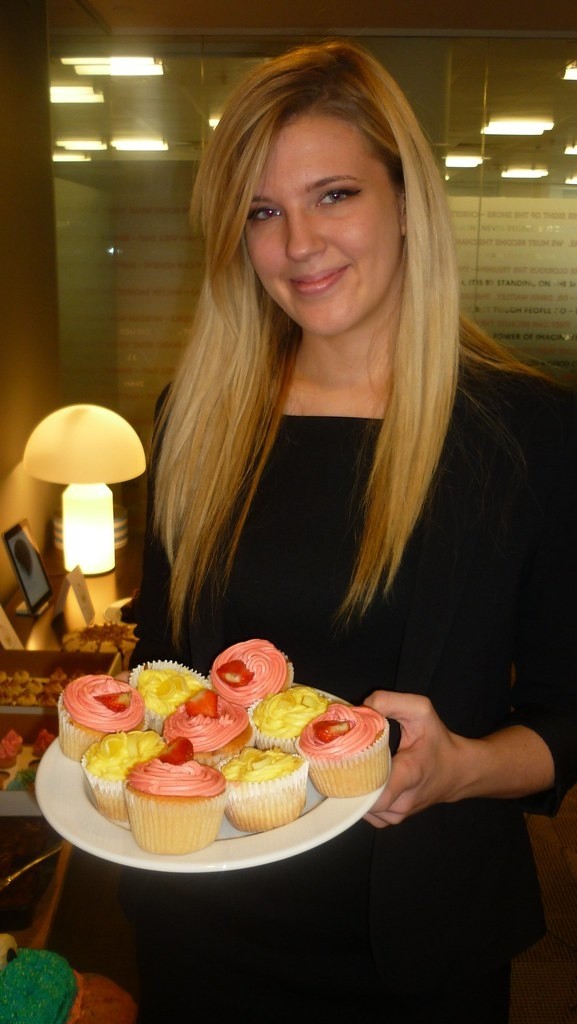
[0, 636, 392, 857]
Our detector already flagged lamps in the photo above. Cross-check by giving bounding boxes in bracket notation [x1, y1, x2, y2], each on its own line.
[49, 56, 577, 187]
[20, 402, 147, 576]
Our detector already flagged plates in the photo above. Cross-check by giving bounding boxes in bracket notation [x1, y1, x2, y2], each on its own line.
[34, 680, 392, 874]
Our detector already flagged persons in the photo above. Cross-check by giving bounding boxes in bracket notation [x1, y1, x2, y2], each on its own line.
[116, 42, 575, 1022]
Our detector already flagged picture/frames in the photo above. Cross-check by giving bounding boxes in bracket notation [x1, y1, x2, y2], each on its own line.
[2, 519, 54, 615]
